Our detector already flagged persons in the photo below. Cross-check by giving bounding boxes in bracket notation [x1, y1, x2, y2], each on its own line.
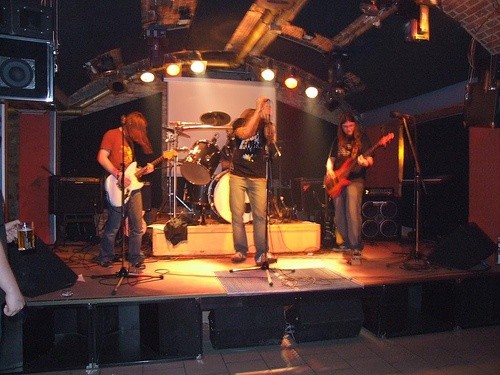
[231, 96, 278, 265]
[97, 111, 154, 268]
[327, 114, 374, 255]
[221, 129, 235, 170]
[0, 242, 25, 316]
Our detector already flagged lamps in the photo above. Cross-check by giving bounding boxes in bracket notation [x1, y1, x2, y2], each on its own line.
[258, 56, 343, 113]
[397, 0, 442, 42]
[109, 58, 208, 93]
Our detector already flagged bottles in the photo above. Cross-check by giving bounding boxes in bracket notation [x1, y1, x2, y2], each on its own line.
[495, 236, 500, 265]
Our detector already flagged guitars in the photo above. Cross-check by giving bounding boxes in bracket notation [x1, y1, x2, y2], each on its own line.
[103, 149, 179, 208]
[323, 132, 395, 198]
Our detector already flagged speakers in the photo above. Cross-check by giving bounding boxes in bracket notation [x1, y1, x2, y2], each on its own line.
[0, 32, 56, 105]
[59, 175, 101, 213]
[291, 177, 328, 232]
[360, 191, 399, 243]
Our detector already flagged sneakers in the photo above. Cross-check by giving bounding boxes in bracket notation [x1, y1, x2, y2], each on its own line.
[231, 252, 246, 261]
[256, 252, 277, 266]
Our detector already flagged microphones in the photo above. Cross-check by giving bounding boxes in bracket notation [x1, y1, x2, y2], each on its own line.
[389, 111, 403, 116]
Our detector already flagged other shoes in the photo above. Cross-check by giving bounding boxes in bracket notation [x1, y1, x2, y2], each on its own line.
[132, 262, 143, 269]
[353, 248, 361, 258]
[101, 256, 112, 266]
[333, 245, 351, 252]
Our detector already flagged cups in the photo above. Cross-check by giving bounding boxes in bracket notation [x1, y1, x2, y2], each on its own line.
[15, 221, 35, 250]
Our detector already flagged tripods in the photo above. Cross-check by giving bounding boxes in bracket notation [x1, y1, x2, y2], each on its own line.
[92, 119, 164, 296]
[385, 116, 443, 271]
[152, 130, 195, 221]
[229, 100, 296, 287]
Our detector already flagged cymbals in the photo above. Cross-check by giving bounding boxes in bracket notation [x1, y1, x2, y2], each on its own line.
[200, 110, 232, 127]
[160, 126, 191, 139]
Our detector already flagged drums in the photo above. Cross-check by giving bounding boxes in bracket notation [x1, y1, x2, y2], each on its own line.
[180, 138, 222, 186]
[206, 168, 254, 225]
